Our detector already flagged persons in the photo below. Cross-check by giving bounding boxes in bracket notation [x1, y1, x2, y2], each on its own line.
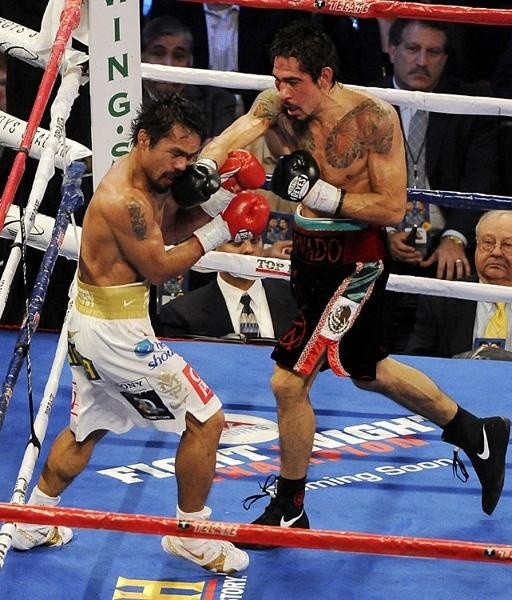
[6, 88, 250, 576]
[137, 1, 511, 361]
[169, 23, 511, 553]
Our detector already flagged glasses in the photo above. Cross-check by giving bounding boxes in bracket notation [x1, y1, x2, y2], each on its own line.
[475, 238, 512, 252]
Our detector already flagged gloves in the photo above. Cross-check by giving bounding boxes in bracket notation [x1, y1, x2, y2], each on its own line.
[192, 191, 270, 254]
[271, 151, 342, 216]
[172, 159, 221, 207]
[200, 149, 267, 217]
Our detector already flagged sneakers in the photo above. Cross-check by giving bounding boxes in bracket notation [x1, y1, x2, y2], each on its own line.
[473, 414, 511, 515]
[161, 536, 250, 575]
[13, 524, 73, 549]
[236, 499, 312, 550]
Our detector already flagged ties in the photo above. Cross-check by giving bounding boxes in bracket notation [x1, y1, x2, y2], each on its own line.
[240, 295, 258, 336]
[407, 111, 427, 189]
[483, 301, 508, 339]
